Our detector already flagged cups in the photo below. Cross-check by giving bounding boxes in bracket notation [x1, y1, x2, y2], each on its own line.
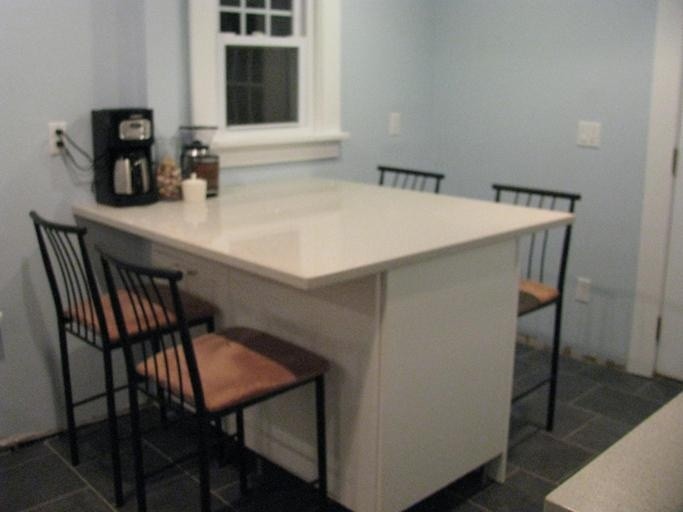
[180, 140, 220, 203]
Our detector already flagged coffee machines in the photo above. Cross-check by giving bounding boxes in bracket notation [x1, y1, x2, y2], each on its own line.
[92, 107, 159, 207]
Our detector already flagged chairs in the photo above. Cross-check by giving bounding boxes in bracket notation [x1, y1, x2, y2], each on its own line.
[492, 184, 581, 432]
[95, 244, 331, 512]
[378, 166, 444, 193]
[30, 210, 220, 510]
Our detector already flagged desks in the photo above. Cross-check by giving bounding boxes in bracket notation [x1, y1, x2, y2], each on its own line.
[543, 393, 682, 512]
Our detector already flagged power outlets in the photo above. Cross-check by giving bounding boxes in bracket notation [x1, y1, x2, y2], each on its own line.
[48, 121, 68, 156]
